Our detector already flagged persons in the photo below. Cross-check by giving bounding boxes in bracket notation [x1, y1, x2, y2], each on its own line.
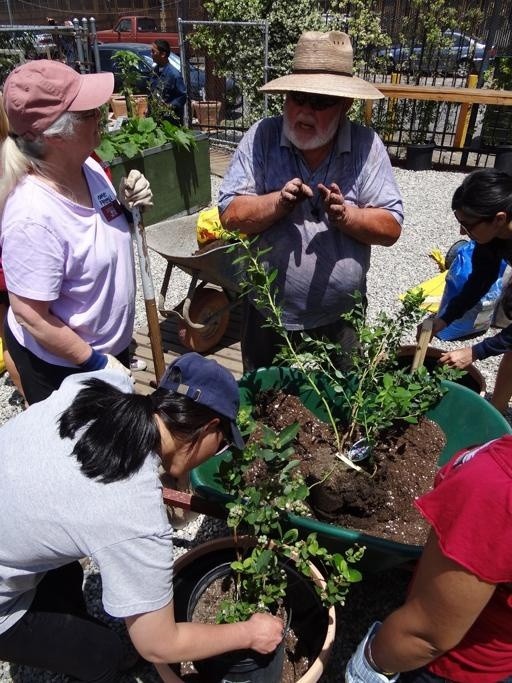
[1, 56, 153, 404]
[146, 37, 188, 129]
[47, 16, 81, 71]
[415, 167, 511, 411]
[215, 27, 404, 402]
[345, 434, 512, 683]
[0, 352, 285, 683]
[0, 93, 113, 410]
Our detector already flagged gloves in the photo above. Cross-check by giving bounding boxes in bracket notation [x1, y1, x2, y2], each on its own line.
[117, 170, 154, 223]
[80, 349, 136, 384]
[344, 622, 403, 683]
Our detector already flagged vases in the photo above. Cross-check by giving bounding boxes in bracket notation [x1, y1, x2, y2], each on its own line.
[194, 100, 221, 130]
[111, 95, 147, 120]
[381, 346, 487, 398]
[109, 93, 120, 104]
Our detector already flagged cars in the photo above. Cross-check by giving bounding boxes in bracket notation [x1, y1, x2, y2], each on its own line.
[372, 31, 498, 78]
[68, 43, 244, 119]
[0, 33, 63, 60]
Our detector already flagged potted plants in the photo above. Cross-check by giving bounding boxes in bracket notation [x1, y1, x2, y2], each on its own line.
[214, 220, 470, 521]
[183, 401, 366, 683]
[405, 100, 441, 170]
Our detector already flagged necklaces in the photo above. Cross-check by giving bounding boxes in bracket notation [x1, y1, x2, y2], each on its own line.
[295, 131, 338, 223]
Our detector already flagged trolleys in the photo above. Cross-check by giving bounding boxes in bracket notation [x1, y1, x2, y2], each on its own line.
[141, 205, 255, 371]
[150, 365, 511, 575]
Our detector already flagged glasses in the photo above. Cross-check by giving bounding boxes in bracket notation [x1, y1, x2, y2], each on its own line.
[451, 212, 486, 234]
[290, 90, 345, 111]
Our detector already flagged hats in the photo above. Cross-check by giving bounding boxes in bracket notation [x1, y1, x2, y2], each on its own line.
[3, 59, 115, 143]
[260, 31, 386, 99]
[158, 353, 246, 449]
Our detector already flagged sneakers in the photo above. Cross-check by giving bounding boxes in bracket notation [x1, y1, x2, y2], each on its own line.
[128, 353, 147, 371]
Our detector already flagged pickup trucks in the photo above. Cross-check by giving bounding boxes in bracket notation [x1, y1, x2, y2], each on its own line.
[89, 16, 193, 57]
